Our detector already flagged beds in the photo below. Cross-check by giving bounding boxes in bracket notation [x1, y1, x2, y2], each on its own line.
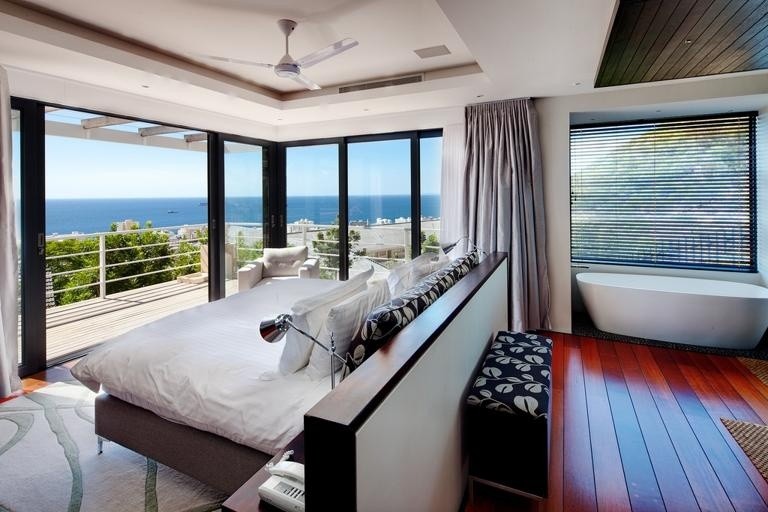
[70, 252, 511, 510]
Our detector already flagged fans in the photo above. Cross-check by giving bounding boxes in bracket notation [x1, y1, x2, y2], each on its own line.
[209, 18, 359, 91]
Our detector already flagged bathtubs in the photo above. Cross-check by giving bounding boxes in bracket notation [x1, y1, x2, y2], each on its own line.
[575, 272, 767, 349]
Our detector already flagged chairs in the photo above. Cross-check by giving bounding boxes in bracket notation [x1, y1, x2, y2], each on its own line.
[237, 246, 319, 292]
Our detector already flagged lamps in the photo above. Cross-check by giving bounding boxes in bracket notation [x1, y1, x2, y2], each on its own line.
[432, 237, 479, 260]
[260, 313, 347, 389]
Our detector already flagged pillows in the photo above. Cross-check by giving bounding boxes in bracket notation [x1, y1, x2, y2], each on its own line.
[307, 278, 390, 382]
[408, 256, 450, 287]
[341, 250, 479, 377]
[279, 266, 374, 374]
[389, 251, 453, 294]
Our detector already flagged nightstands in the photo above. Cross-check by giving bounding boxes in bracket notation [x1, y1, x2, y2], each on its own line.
[220, 431, 305, 512]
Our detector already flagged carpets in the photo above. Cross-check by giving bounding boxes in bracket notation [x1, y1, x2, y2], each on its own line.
[737, 356, 768, 387]
[720, 417, 768, 485]
[0, 379, 230, 512]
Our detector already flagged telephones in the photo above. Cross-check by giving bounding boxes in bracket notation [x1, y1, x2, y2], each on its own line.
[257, 461, 305, 512]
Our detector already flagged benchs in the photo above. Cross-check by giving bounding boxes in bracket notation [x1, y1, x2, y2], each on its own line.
[463, 331, 554, 512]
